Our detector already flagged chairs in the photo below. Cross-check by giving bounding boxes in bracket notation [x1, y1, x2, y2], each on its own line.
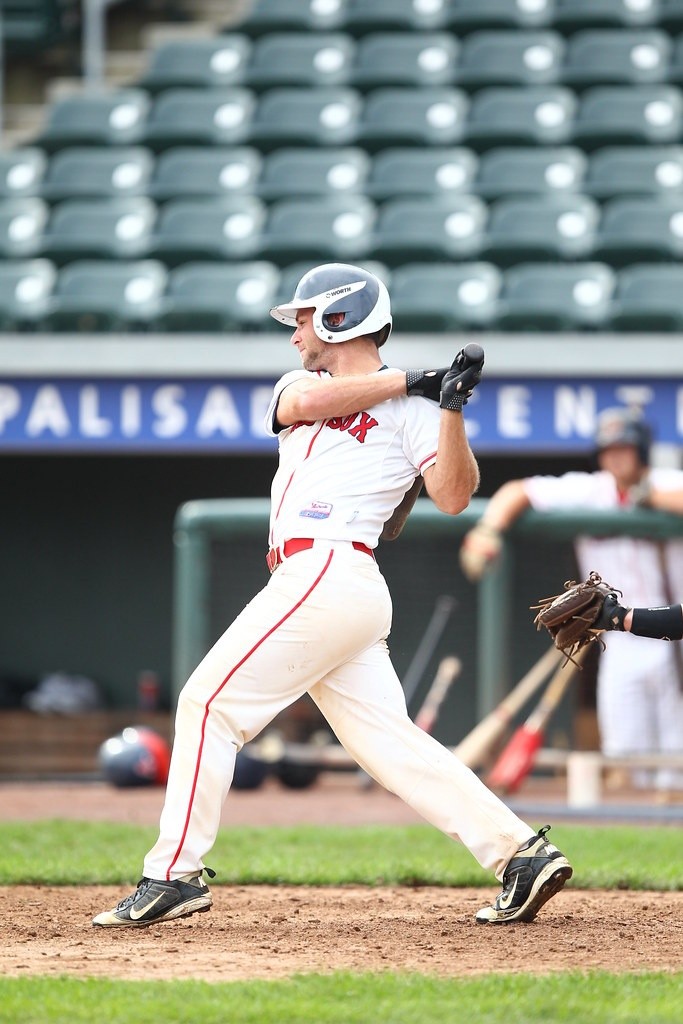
[0, 1, 683, 335]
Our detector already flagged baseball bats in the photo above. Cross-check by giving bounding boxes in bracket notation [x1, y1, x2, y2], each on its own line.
[382, 342, 486, 541]
[415, 632, 597, 796]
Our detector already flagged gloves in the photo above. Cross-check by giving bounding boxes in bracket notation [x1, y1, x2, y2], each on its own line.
[440, 350, 482, 412]
[407, 368, 474, 405]
[459, 521, 501, 581]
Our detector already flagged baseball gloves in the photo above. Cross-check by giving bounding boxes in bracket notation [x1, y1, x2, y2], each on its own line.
[532, 569, 623, 672]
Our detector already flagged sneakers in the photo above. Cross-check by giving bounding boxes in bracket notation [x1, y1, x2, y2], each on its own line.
[476, 838, 572, 924]
[91, 869, 213, 928]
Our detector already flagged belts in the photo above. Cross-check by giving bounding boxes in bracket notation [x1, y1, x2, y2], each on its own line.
[265, 538, 375, 573]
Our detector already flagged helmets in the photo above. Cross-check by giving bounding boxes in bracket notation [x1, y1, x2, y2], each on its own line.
[594, 407, 652, 453]
[271, 263, 392, 345]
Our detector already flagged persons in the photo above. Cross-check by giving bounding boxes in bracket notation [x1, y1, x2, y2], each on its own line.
[587, 596, 683, 641]
[459, 420, 682, 805]
[92, 263, 573, 928]
